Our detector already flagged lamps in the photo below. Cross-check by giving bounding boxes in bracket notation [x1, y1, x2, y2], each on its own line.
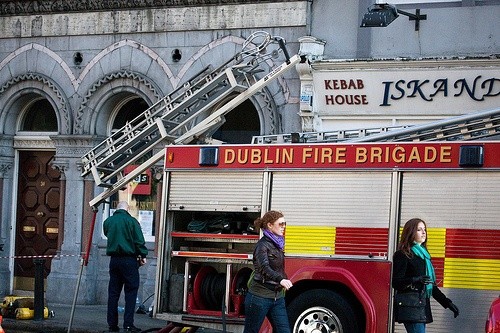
[172, 49, 182, 62]
[360, 3, 427, 31]
[73, 52, 83, 65]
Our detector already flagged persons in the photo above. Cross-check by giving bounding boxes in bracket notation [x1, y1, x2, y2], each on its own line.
[103, 201, 148, 333]
[244, 211, 293, 333]
[392, 218, 459, 333]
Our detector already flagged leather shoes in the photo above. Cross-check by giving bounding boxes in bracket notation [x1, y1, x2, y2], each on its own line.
[124, 324, 141, 331]
[109, 326, 120, 332]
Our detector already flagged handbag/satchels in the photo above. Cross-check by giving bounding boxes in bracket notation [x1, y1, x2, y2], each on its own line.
[396, 292, 425, 321]
[247, 270, 256, 289]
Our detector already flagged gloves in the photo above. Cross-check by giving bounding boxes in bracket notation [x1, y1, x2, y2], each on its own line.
[448, 303, 459, 318]
[412, 280, 424, 291]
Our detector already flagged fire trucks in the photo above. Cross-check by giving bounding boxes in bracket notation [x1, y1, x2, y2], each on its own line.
[80, 31, 500, 333]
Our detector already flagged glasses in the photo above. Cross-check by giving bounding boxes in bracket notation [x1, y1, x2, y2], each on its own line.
[272, 222, 286, 227]
